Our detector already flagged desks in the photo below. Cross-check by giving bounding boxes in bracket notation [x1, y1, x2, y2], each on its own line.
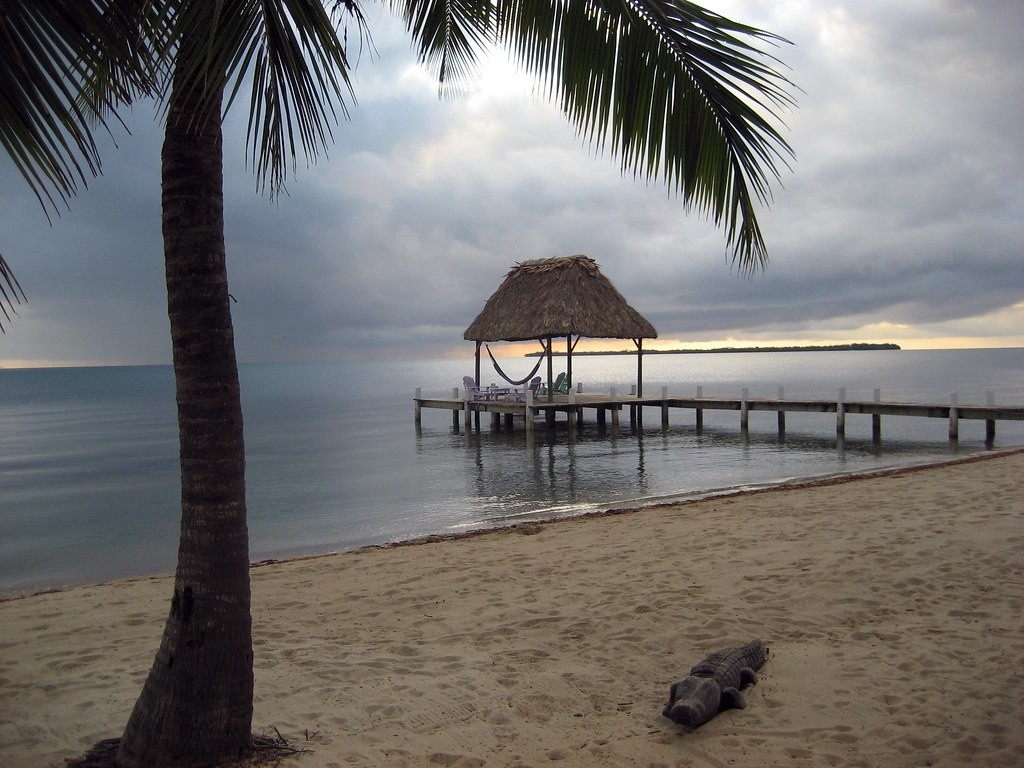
[490, 387, 511, 401]
[540, 382, 548, 387]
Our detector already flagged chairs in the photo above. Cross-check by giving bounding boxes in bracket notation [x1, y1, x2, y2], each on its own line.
[463, 372, 569, 401]
